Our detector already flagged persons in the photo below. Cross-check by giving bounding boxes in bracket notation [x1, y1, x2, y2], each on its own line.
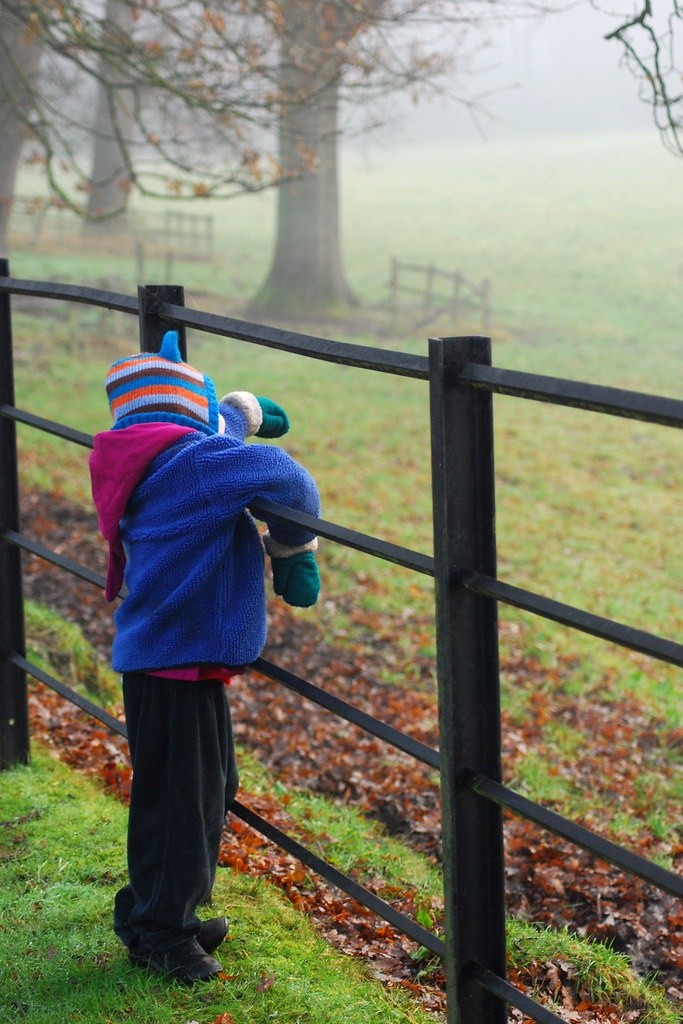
[87, 328, 321, 981]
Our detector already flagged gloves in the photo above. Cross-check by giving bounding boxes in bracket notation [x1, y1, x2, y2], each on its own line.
[221, 392, 290, 439]
[262, 531, 321, 607]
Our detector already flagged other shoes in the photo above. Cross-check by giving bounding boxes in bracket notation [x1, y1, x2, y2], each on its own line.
[129, 916, 228, 968]
[154, 936, 222, 984]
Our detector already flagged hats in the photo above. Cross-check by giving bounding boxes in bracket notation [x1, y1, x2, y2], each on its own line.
[105, 331, 219, 434]
